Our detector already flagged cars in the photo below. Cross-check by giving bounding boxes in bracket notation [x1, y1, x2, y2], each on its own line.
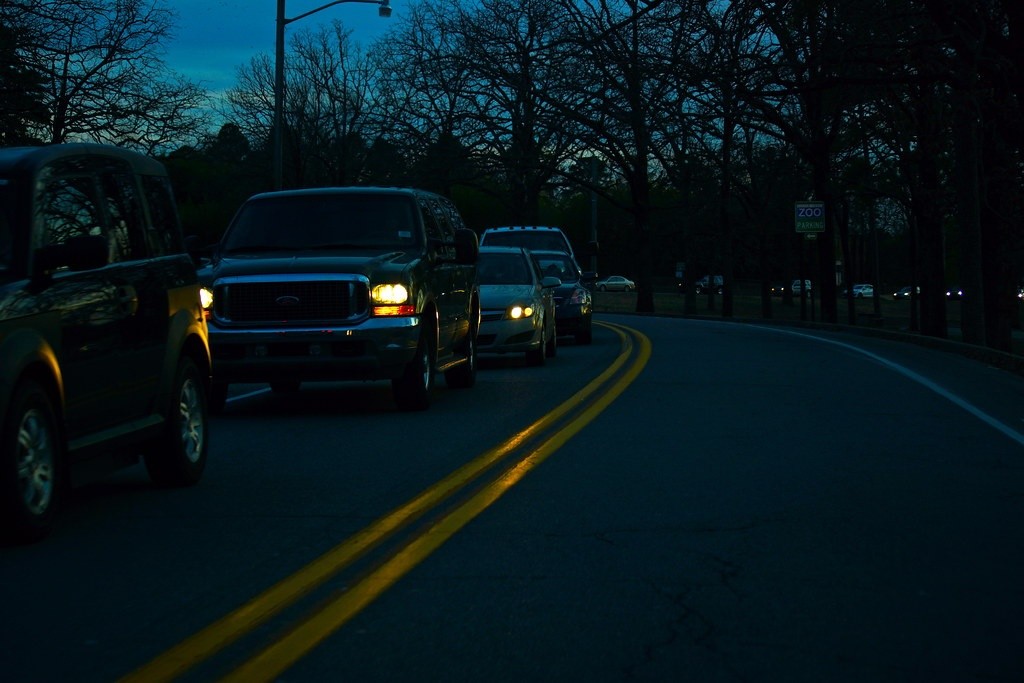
[477, 224, 598, 368]
[596, 274, 636, 293]
[843, 283, 874, 302]
[893, 285, 920, 301]
[769, 283, 786, 296]
[942, 279, 966, 300]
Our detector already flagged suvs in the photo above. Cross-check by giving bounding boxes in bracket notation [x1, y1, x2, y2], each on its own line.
[0, 141, 216, 524]
[197, 185, 483, 411]
[695, 274, 724, 296]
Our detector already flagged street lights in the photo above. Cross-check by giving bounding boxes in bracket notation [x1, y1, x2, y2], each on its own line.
[268, 0, 392, 181]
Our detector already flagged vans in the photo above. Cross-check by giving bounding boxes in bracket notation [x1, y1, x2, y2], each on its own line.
[789, 279, 814, 299]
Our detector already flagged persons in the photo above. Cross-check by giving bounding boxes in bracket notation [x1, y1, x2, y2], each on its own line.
[546, 264, 561, 278]
